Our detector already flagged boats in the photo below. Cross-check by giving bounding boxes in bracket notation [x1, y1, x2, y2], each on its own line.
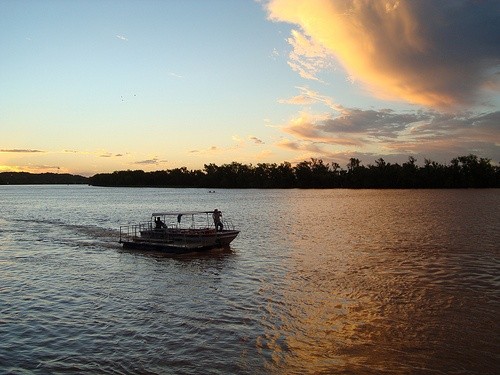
[118, 209, 240, 258]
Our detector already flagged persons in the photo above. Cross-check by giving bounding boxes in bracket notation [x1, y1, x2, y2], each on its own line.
[154, 216, 164, 232]
[212, 209, 223, 232]
